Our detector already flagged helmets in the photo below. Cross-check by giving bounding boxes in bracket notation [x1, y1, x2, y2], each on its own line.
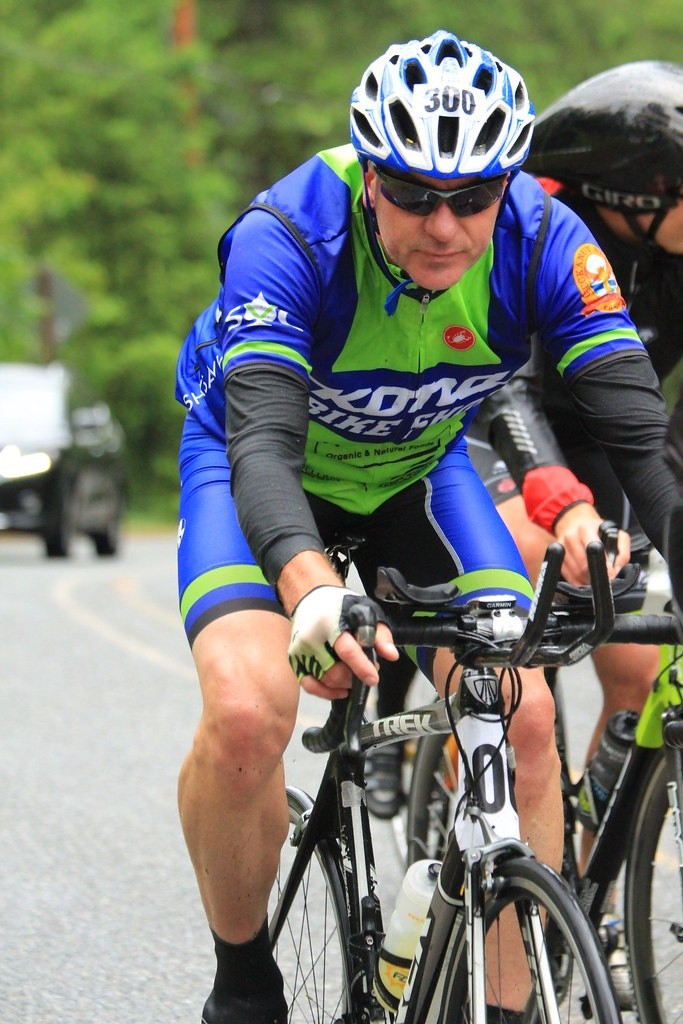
[517, 58, 683, 193]
[350, 31, 534, 180]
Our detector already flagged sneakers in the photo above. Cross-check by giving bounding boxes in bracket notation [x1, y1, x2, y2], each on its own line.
[598, 915, 637, 1009]
[360, 743, 405, 817]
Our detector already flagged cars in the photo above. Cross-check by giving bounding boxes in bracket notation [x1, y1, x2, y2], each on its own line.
[1, 357, 128, 558]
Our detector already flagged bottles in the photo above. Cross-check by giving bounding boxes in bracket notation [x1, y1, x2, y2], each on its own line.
[372, 859, 445, 1014]
[577, 710, 642, 834]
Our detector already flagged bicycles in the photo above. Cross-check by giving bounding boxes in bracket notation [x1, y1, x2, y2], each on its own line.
[263, 521, 682, 1023]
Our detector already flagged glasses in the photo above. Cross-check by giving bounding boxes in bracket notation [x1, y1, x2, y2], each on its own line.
[367, 161, 509, 218]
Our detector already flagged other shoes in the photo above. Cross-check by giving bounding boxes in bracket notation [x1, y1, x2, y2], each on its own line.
[202, 988, 287, 1024]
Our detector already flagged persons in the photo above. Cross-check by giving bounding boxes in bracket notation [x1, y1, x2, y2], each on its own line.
[364, 57, 683, 1013]
[172, 32, 683, 1024]
